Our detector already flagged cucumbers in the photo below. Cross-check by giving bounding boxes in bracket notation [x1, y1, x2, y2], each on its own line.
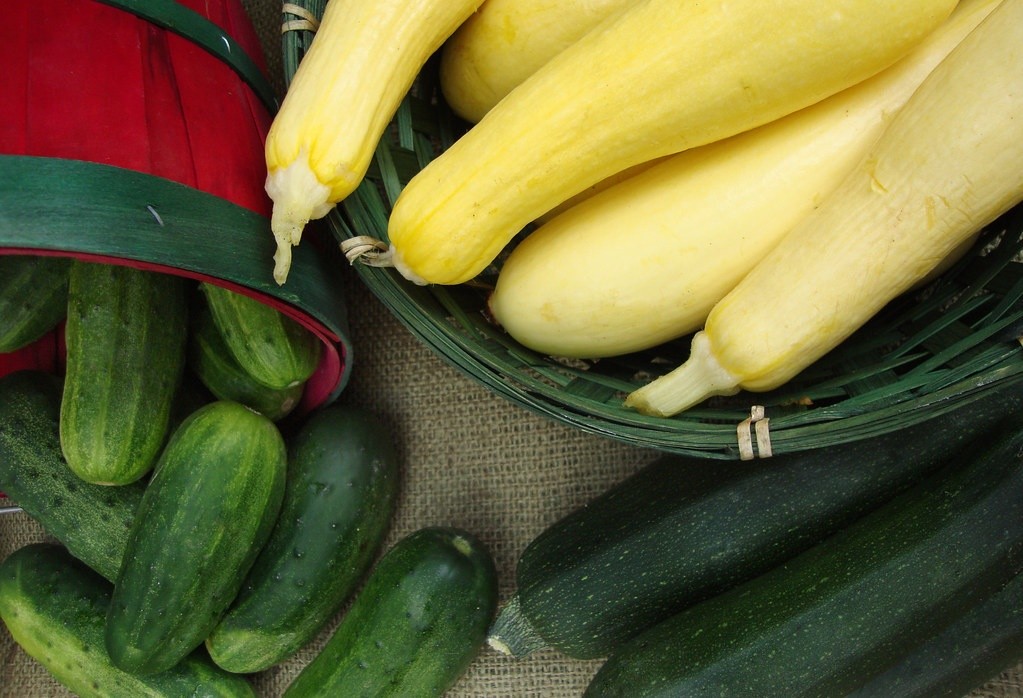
[0, 240, 494, 697]
[498, 432, 1023, 698]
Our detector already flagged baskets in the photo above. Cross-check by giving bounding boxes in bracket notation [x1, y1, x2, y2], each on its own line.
[281, 0, 1023, 465]
